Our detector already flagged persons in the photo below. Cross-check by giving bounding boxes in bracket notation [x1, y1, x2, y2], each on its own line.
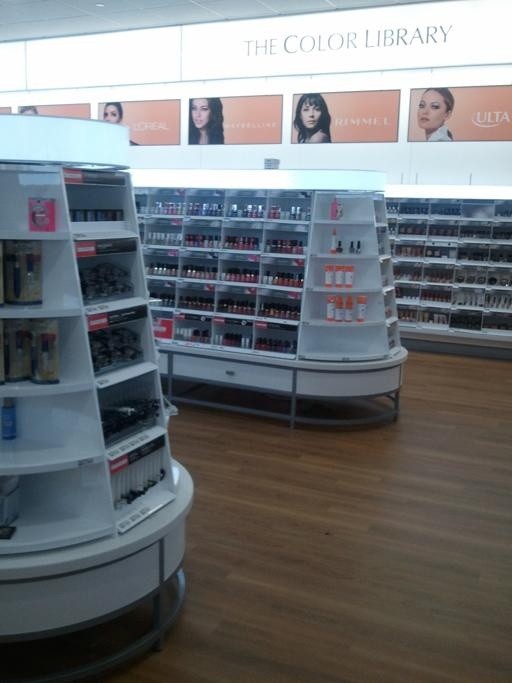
[189, 98, 224, 144]
[292, 93, 332, 143]
[103, 102, 138, 146]
[417, 88, 456, 141]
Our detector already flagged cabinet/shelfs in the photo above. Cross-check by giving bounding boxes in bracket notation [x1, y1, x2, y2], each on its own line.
[126, 183, 409, 431]
[383, 195, 511, 360]
[1, 164, 197, 681]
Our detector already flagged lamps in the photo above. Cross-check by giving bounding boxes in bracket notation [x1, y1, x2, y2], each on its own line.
[0, 112, 132, 169]
[129, 164, 391, 194]
[381, 181, 511, 200]
